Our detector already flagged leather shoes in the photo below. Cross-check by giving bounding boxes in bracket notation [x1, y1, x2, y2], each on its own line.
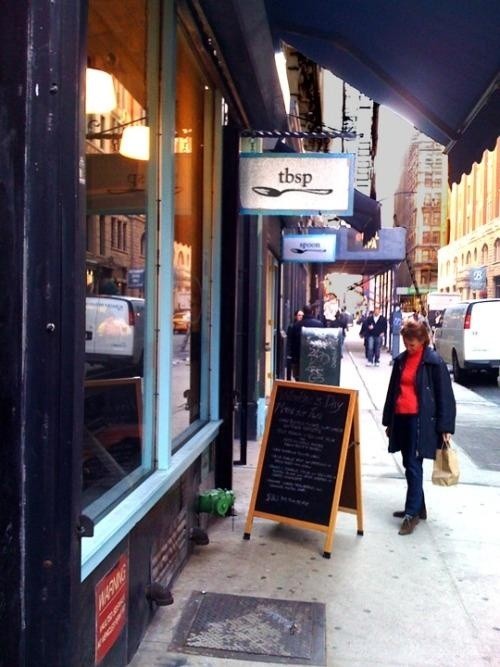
[392, 508, 428, 536]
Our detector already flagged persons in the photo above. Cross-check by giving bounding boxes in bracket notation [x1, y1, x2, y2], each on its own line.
[285, 304, 388, 380]
[100, 268, 122, 295]
[382, 320, 457, 534]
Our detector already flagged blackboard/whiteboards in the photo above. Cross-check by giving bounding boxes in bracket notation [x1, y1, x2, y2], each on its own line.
[250, 379, 357, 534]
[299, 326, 343, 387]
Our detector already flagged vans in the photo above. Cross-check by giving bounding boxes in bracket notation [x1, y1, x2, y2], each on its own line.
[434, 297, 500, 386]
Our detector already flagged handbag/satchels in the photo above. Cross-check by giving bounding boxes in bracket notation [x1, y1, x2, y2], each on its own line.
[430, 440, 462, 487]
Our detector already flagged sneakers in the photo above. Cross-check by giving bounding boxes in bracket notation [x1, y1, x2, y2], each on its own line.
[365, 361, 380, 368]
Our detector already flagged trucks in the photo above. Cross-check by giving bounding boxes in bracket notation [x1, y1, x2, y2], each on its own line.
[425, 293, 461, 337]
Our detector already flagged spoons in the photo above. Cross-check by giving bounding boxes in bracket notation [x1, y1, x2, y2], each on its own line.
[251, 185, 334, 197]
[290, 247, 327, 254]
